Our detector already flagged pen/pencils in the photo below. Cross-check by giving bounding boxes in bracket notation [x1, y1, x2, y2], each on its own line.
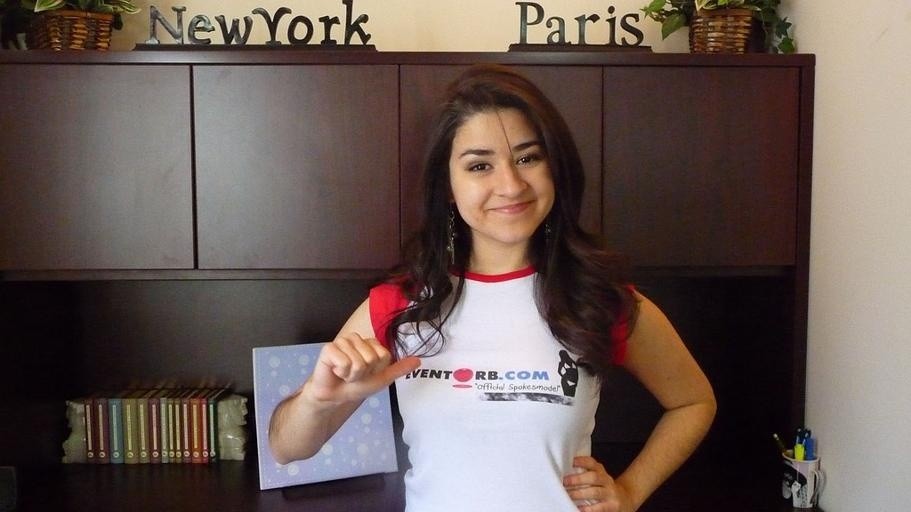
[773, 429, 815, 460]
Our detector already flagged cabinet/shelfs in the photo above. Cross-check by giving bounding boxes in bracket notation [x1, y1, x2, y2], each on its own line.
[397, 52, 814, 281]
[3, 51, 397, 281]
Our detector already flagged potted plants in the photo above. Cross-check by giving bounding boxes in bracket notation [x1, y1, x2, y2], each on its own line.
[636, 0, 805, 60]
[0, 0, 144, 58]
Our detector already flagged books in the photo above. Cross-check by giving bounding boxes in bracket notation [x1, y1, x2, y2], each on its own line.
[83, 387, 228, 465]
[252, 344, 404, 493]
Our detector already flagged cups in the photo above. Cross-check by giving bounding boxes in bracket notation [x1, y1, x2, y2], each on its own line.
[778, 450, 823, 510]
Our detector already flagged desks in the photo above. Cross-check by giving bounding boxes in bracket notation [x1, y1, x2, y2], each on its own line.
[0, 412, 414, 511]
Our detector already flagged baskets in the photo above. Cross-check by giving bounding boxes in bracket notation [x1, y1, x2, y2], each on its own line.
[23, 9, 114, 51]
[687, 7, 753, 53]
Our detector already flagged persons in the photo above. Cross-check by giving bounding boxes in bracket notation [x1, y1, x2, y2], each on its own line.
[267, 66, 719, 512]
